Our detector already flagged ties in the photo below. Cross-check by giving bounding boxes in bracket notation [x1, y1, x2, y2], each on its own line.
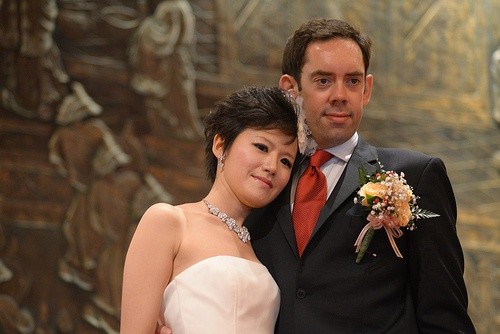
[289, 150, 335, 257]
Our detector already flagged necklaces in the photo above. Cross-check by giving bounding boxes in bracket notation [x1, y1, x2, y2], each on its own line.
[203, 198, 251, 243]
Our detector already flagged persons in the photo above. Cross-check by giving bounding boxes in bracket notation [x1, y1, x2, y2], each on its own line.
[156, 19, 476, 334]
[120, 87, 312, 334]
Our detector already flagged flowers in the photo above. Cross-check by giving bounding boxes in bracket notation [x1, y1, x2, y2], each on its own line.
[349, 161, 440, 262]
[286, 92, 318, 157]
